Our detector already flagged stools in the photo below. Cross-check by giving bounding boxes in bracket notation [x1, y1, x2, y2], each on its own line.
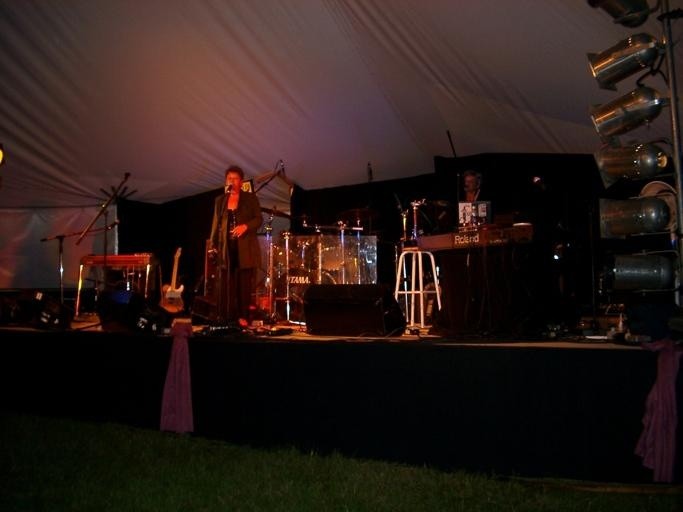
[394, 250, 442, 326]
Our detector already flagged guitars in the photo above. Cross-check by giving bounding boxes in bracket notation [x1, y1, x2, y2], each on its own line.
[159, 248, 185, 314]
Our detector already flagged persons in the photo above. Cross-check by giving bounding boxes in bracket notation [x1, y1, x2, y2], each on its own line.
[463, 170, 486, 202]
[209, 166, 262, 324]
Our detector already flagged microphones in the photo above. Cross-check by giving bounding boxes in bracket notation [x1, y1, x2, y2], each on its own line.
[108, 220, 120, 230]
[226, 183, 233, 193]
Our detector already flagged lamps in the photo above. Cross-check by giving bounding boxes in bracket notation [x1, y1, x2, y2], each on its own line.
[587, 0, 681, 306]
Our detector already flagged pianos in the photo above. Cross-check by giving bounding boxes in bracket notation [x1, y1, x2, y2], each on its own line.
[81, 251, 159, 272]
[416, 223, 533, 252]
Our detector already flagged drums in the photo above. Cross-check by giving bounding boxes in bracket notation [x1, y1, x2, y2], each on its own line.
[266, 247, 358, 326]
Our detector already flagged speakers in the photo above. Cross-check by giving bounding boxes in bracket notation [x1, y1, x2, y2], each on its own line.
[94, 283, 172, 330]
[304, 284, 407, 338]
[14, 292, 72, 326]
